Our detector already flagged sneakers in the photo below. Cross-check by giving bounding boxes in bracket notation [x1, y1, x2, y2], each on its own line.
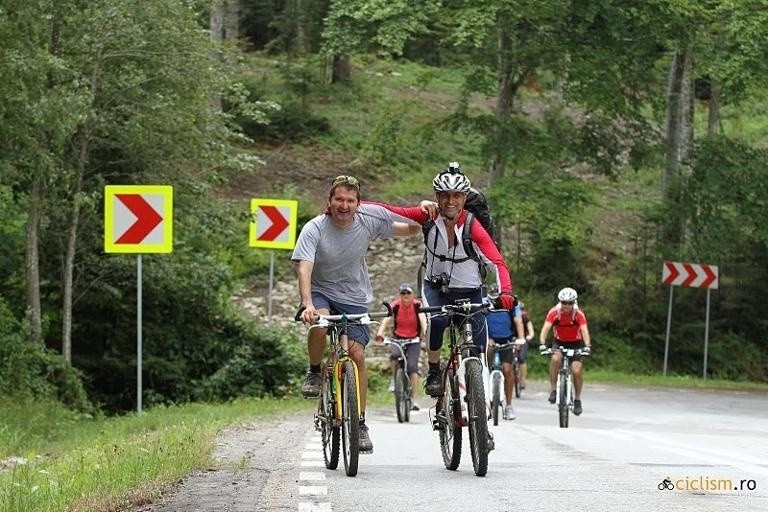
[388, 380, 395, 393]
[301, 371, 323, 397]
[520, 380, 525, 389]
[549, 391, 556, 403]
[422, 369, 445, 397]
[574, 399, 582, 416]
[505, 406, 516, 420]
[412, 403, 420, 410]
[358, 424, 373, 451]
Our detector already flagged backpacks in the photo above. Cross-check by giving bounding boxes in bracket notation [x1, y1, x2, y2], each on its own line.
[423, 187, 499, 268]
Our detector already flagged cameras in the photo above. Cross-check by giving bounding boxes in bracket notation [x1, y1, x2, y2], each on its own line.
[431, 275, 451, 297]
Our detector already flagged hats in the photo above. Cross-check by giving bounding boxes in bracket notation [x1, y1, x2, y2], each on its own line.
[400, 283, 413, 292]
[330, 175, 361, 192]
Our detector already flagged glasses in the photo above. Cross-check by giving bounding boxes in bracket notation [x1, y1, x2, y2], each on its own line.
[561, 301, 575, 305]
[400, 291, 412, 294]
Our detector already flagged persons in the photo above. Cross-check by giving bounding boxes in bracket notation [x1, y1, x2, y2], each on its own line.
[515, 301, 535, 390]
[374, 283, 429, 412]
[289, 174, 441, 453]
[322, 170, 516, 453]
[538, 287, 592, 416]
[478, 281, 527, 420]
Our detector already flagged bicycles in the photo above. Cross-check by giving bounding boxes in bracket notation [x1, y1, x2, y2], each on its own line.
[414, 295, 517, 476]
[295, 301, 393, 476]
[481, 341, 525, 426]
[512, 337, 527, 398]
[538, 346, 589, 427]
[372, 334, 426, 423]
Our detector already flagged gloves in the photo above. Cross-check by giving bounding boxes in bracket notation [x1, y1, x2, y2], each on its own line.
[582, 346, 592, 356]
[539, 344, 547, 355]
[496, 293, 515, 311]
[375, 336, 384, 343]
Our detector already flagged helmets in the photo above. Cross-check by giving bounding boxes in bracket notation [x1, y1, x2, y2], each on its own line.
[433, 171, 471, 194]
[486, 284, 500, 300]
[558, 287, 578, 302]
